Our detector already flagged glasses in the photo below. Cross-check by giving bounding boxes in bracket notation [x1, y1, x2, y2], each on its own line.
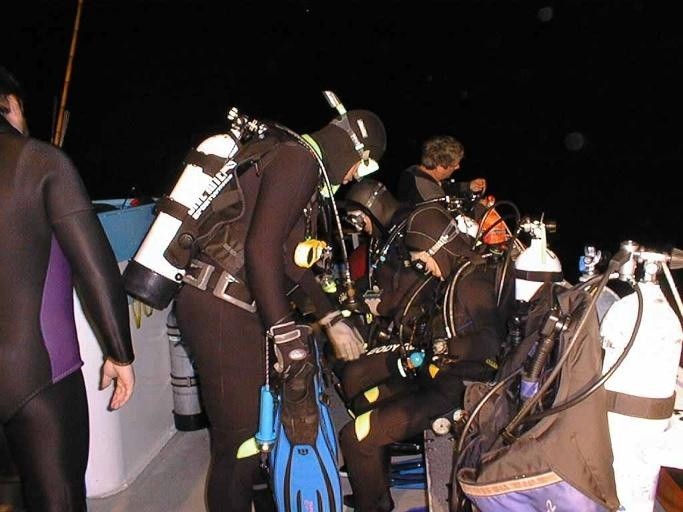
[322, 90, 380, 183]
[344, 182, 387, 232]
[411, 218, 460, 274]
[450, 164, 460, 171]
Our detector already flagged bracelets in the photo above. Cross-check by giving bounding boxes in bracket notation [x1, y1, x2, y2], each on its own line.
[320, 313, 347, 331]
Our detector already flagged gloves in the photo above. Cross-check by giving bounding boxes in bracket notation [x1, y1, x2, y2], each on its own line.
[317, 310, 367, 361]
[364, 297, 383, 316]
[270, 321, 309, 378]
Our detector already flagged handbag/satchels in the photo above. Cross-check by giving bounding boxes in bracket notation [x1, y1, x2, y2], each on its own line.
[447, 283, 622, 512]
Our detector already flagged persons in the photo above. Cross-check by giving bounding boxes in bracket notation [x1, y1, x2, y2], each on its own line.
[282, 138, 516, 512]
[0, 70, 135, 512]
[174, 110, 388, 511]
